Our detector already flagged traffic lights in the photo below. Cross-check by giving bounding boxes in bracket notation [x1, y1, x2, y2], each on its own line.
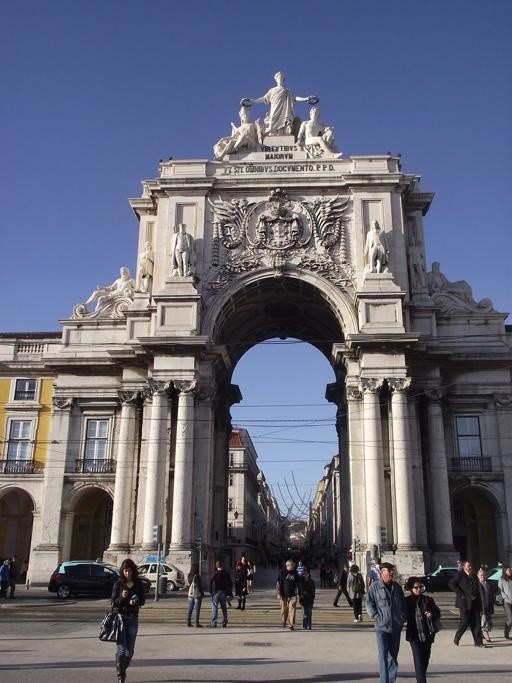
[345, 550, 353, 562]
[152, 524, 163, 544]
[377, 526, 387, 545]
[195, 535, 203, 551]
[355, 537, 361, 552]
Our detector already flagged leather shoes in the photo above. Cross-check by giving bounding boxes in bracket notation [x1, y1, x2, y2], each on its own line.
[475, 643, 486, 648]
[453, 637, 459, 646]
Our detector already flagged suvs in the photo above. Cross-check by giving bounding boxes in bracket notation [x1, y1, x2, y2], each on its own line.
[47, 558, 151, 600]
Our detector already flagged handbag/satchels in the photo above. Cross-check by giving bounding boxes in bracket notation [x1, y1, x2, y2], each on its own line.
[188, 575, 202, 599]
[422, 596, 441, 634]
[98, 602, 128, 641]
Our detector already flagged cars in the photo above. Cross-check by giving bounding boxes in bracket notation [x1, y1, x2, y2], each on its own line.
[405, 567, 460, 593]
[484, 566, 505, 607]
[134, 561, 186, 592]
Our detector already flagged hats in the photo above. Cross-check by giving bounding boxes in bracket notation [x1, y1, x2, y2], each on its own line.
[379, 562, 394, 570]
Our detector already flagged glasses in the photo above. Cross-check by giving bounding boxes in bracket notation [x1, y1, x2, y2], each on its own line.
[413, 586, 422, 589]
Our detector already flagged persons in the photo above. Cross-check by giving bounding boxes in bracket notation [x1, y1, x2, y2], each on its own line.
[214, 71, 343, 160]
[364, 219, 389, 273]
[82, 266, 135, 311]
[447, 559, 512, 648]
[170, 223, 192, 277]
[408, 236, 425, 288]
[0, 555, 28, 599]
[111, 558, 144, 683]
[186, 563, 204, 627]
[276, 559, 315, 631]
[136, 240, 154, 291]
[427, 261, 475, 304]
[332, 558, 440, 683]
[206, 555, 256, 628]
[319, 558, 328, 588]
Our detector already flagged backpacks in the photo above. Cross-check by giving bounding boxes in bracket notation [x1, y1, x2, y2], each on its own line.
[351, 573, 364, 593]
